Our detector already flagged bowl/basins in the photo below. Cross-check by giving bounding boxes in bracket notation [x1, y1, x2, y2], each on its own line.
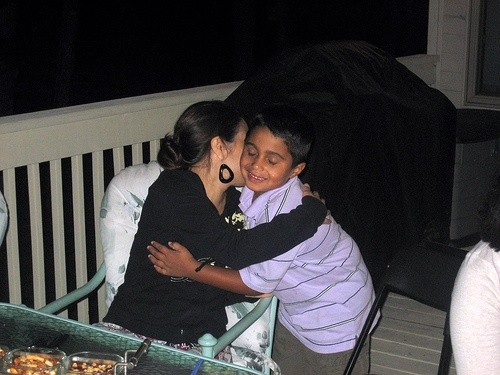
[45, 351, 124, 375]
[0, 345, 9, 359]
[0, 346, 66, 375]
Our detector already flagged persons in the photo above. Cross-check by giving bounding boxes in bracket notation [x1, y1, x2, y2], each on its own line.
[99, 100, 331, 375]
[146, 108, 381, 375]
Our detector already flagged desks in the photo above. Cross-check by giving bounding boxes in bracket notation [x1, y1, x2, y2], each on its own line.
[0, 301, 265, 375]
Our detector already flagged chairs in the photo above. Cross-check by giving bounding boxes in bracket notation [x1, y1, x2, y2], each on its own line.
[343, 241, 469, 375]
[35, 162, 279, 375]
